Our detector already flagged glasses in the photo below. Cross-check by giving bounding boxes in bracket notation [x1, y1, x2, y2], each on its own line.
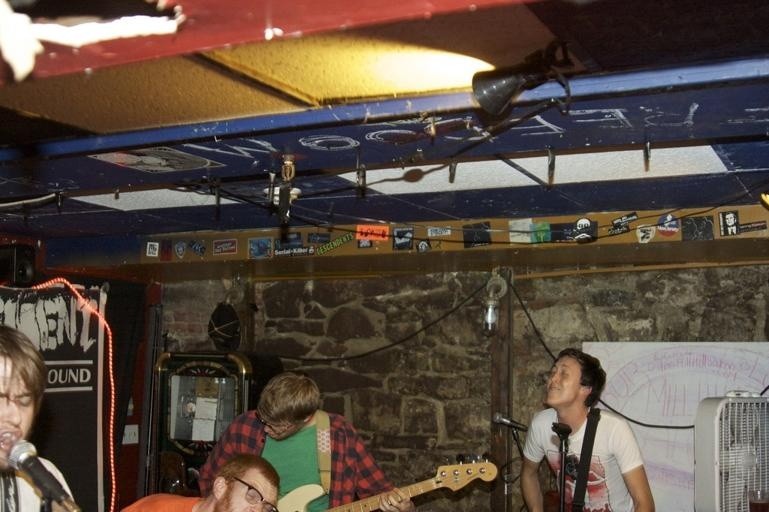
[255, 411, 293, 435]
[232, 475, 279, 511]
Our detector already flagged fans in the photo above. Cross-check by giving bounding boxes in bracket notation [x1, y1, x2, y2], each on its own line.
[695, 397, 769, 511]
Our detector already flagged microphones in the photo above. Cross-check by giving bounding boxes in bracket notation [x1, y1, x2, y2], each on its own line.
[493, 413, 528, 432]
[7, 439, 82, 512]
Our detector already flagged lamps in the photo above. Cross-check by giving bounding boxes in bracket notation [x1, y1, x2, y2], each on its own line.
[470, 30, 576, 118]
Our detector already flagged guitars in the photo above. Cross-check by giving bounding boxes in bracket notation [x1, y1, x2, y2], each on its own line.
[274, 457, 498, 512]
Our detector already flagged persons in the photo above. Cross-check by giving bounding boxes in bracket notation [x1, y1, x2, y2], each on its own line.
[724, 212, 739, 236]
[682, 214, 715, 242]
[0, 322, 77, 512]
[118, 452, 281, 512]
[520, 348, 656, 512]
[197, 371, 416, 512]
